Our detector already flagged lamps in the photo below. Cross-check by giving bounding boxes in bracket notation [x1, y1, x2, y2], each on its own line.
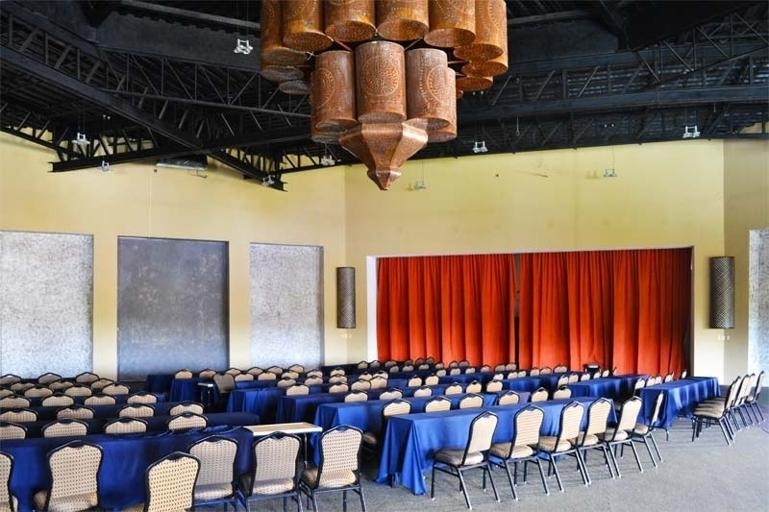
[711, 255, 735, 329]
[259, 1, 513, 192]
[337, 267, 355, 330]
[604, 131, 618, 179]
[683, 126, 700, 139]
[154, 159, 209, 172]
[411, 149, 426, 192]
[99, 160, 113, 174]
[472, 140, 488, 153]
[233, 36, 252, 56]
[70, 130, 91, 145]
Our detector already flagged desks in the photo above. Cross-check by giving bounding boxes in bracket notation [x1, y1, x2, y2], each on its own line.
[374, 396, 618, 498]
[0, 421, 323, 510]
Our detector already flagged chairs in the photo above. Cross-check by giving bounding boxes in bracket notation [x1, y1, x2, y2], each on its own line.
[122, 450, 202, 511]
[1, 369, 209, 439]
[483, 402, 550, 502]
[690, 370, 766, 446]
[146, 363, 573, 461]
[430, 410, 501, 511]
[614, 391, 665, 468]
[242, 430, 304, 512]
[0, 450, 20, 511]
[523, 400, 588, 493]
[33, 439, 105, 512]
[584, 396, 644, 479]
[317, 354, 722, 442]
[186, 434, 241, 512]
[553, 398, 615, 484]
[295, 423, 366, 512]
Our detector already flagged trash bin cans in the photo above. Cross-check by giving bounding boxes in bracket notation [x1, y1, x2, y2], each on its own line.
[582, 364, 600, 379]
